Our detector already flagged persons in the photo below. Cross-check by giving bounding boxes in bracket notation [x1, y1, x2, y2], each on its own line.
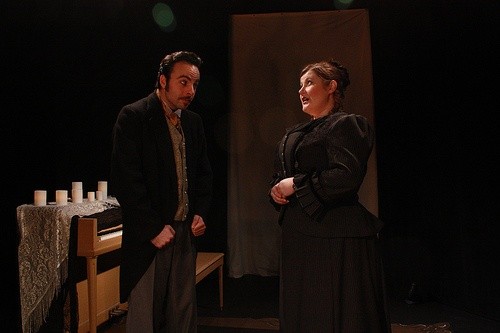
[109, 51, 212, 333]
[267, 59, 389, 333]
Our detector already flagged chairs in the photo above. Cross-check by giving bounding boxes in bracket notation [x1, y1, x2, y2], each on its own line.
[195, 252, 225, 314]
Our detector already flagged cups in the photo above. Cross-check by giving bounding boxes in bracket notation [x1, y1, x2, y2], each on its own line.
[56, 190, 68, 206]
[34, 191, 47, 206]
[88, 192, 95, 201]
[96, 191, 102, 201]
[71, 182, 82, 204]
[98, 181, 107, 201]
